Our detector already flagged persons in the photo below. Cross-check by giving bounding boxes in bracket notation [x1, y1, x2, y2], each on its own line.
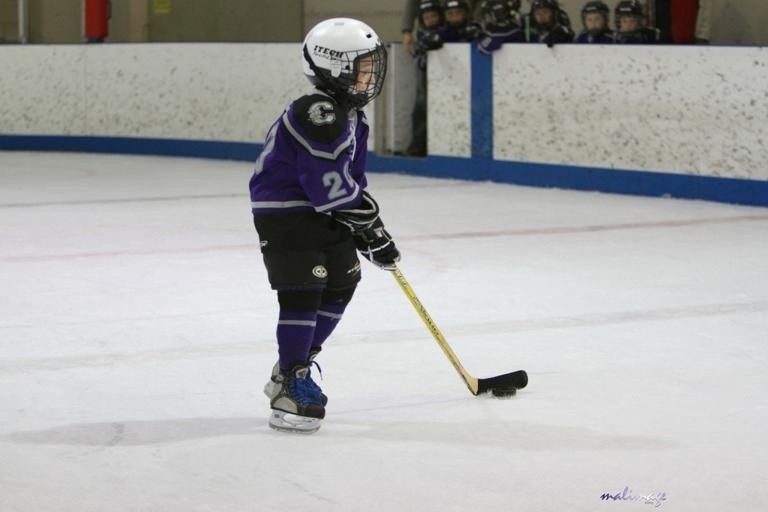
[249, 17, 400, 417]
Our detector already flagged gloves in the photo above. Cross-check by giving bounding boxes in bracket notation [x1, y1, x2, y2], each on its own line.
[332, 191, 379, 238]
[352, 217, 401, 271]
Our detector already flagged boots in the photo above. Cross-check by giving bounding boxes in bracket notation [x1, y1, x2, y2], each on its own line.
[269, 365, 325, 419]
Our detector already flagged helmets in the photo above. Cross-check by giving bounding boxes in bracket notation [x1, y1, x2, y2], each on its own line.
[580, 2, 609, 35]
[479, 1, 510, 23]
[530, 1, 558, 30]
[301, 17, 387, 112]
[439, 0, 469, 28]
[416, 0, 444, 29]
[614, 1, 642, 34]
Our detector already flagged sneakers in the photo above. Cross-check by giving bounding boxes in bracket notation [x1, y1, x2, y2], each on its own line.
[264, 346, 327, 406]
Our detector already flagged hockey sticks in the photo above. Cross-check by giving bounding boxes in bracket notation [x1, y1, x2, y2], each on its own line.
[390, 260, 528, 396]
